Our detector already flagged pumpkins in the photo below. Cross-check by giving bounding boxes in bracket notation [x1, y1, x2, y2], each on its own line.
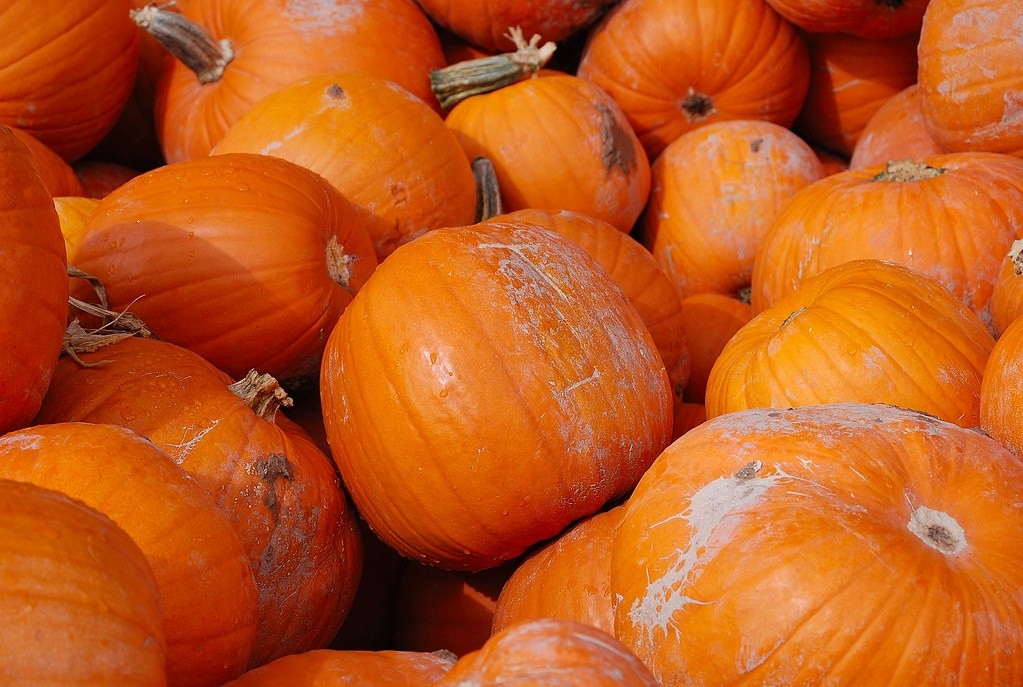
[0, 0, 1023, 687]
